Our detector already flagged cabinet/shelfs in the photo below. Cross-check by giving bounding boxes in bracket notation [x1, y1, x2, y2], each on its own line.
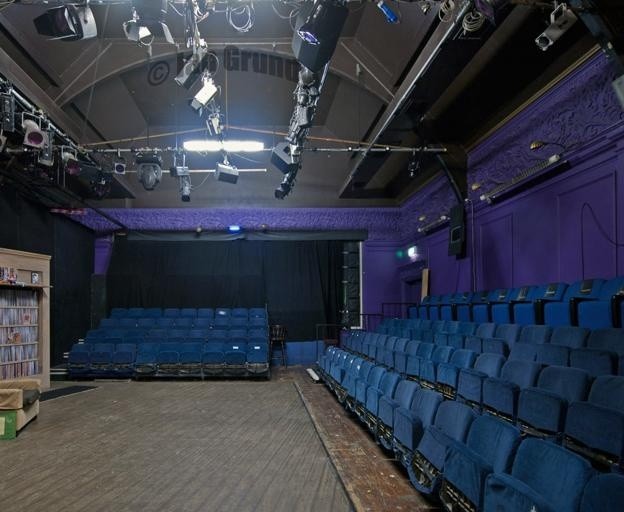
[0, 246, 53, 389]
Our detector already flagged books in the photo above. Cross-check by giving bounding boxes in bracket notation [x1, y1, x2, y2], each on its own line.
[0, 265, 43, 381]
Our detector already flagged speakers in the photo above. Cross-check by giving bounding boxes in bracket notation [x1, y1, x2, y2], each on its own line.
[291, 0, 348, 72]
[270, 141, 292, 174]
[215, 163, 240, 185]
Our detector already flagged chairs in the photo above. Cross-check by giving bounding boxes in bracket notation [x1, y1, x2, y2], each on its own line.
[68, 307, 271, 380]
[318, 277, 624, 511]
[0, 379, 40, 432]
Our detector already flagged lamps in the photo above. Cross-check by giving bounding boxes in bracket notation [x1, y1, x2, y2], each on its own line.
[270, 5, 345, 200]
[410, 155, 419, 179]
[169, 1, 236, 185]
[123, 6, 151, 43]
[535, 11, 574, 53]
[529, 140, 569, 152]
[140, 163, 163, 189]
[2, 81, 126, 196]
[179, 178, 193, 199]
[146, 12, 175, 45]
[470, 177, 502, 191]
